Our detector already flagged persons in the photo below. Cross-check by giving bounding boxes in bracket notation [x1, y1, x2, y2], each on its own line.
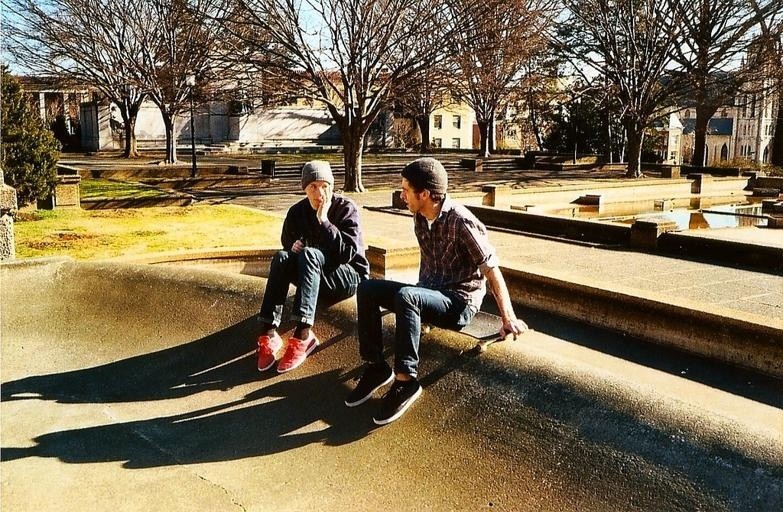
[255, 161, 370, 375]
[344, 157, 529, 425]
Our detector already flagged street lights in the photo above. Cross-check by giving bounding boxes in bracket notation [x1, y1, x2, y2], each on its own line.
[186, 69, 198, 181]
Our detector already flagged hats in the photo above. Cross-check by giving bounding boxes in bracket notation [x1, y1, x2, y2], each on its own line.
[302, 161, 334, 189]
[401, 157, 448, 194]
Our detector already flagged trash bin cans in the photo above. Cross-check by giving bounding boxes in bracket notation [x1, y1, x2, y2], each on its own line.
[261, 160, 274, 178]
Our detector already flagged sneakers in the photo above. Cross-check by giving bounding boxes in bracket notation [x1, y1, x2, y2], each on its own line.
[373, 376, 422, 425]
[277, 329, 319, 373]
[257, 331, 282, 372]
[345, 361, 396, 408]
